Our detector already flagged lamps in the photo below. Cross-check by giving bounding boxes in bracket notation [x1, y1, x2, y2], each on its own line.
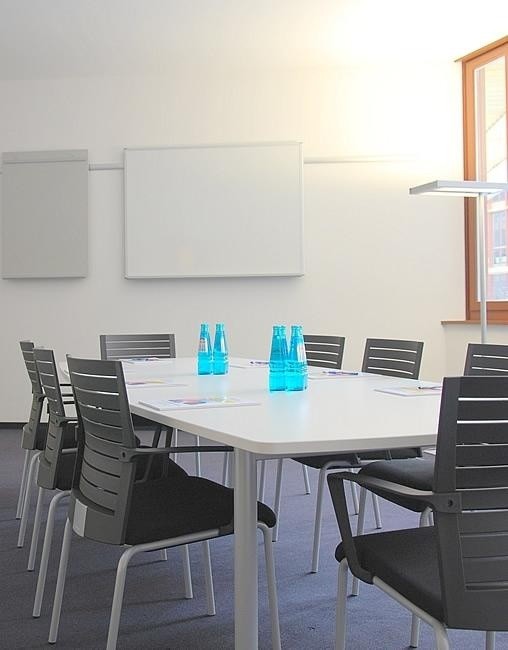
[408, 179, 508, 371]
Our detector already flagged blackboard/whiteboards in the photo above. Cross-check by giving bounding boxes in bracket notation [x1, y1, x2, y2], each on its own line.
[123, 141, 306, 278]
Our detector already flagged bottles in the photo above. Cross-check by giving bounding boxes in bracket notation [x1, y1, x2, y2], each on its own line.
[269, 325, 290, 391]
[287, 326, 309, 391]
[197, 324, 213, 375]
[211, 323, 229, 375]
